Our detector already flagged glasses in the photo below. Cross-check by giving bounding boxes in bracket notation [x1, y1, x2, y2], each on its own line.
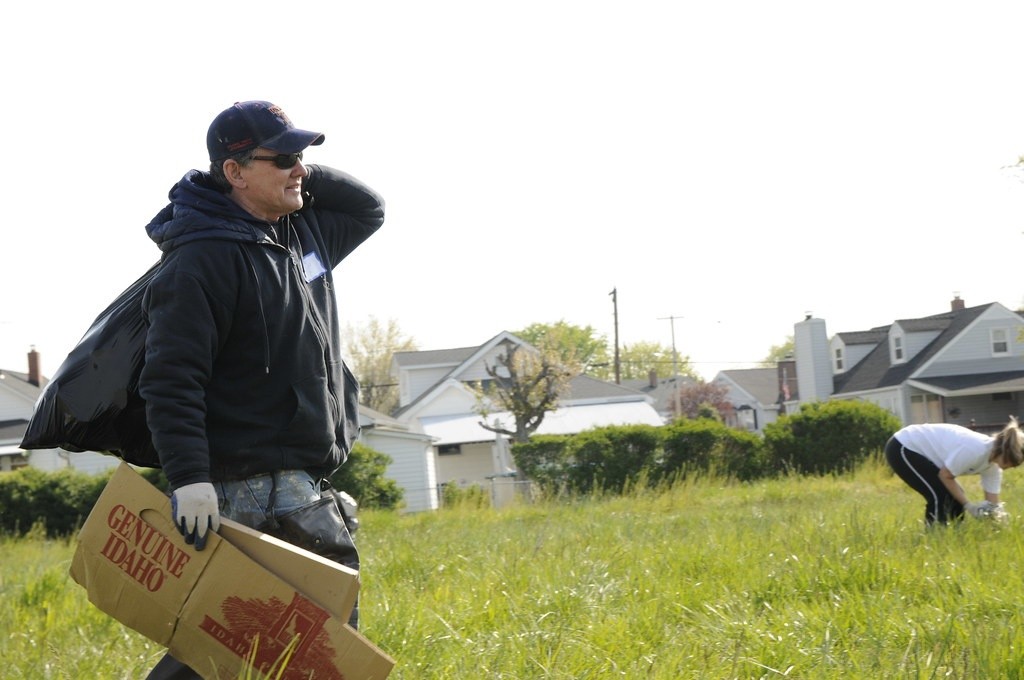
[248, 153, 303, 169]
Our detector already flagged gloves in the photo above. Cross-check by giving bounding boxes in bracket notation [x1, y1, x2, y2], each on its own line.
[990, 503, 1005, 522]
[172, 481, 221, 550]
[964, 501, 988, 522]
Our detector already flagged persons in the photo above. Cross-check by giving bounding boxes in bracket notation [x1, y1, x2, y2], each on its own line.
[883, 414, 1024, 534]
[136, 100, 386, 680]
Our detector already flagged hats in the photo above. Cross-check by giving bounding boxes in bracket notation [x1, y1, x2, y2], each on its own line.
[207, 101, 326, 161]
